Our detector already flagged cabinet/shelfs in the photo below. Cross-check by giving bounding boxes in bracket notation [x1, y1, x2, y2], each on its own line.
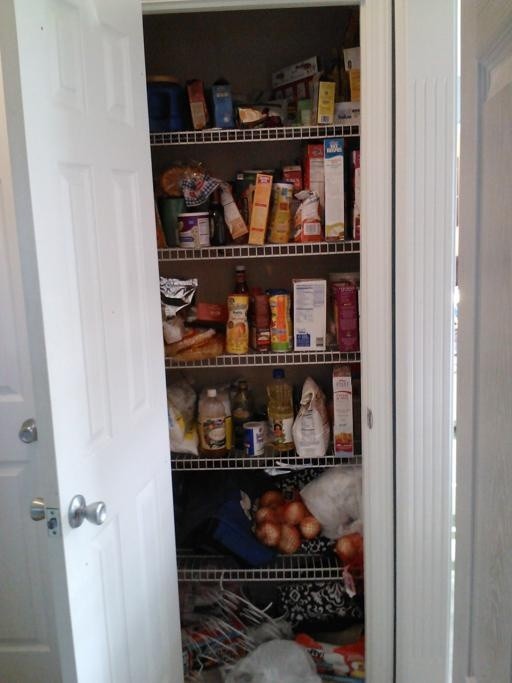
[138, 4, 367, 683]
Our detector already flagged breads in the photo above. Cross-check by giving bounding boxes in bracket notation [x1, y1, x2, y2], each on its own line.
[166, 328, 226, 361]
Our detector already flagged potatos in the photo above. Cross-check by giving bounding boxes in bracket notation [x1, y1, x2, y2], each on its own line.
[253, 492, 321, 554]
[334, 533, 362, 564]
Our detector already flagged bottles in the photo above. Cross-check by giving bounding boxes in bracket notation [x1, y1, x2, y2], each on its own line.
[208, 187, 229, 245]
[225, 265, 293, 356]
[198, 368, 295, 458]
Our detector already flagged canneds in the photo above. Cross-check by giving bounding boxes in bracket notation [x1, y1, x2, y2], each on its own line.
[147, 73, 186, 133]
